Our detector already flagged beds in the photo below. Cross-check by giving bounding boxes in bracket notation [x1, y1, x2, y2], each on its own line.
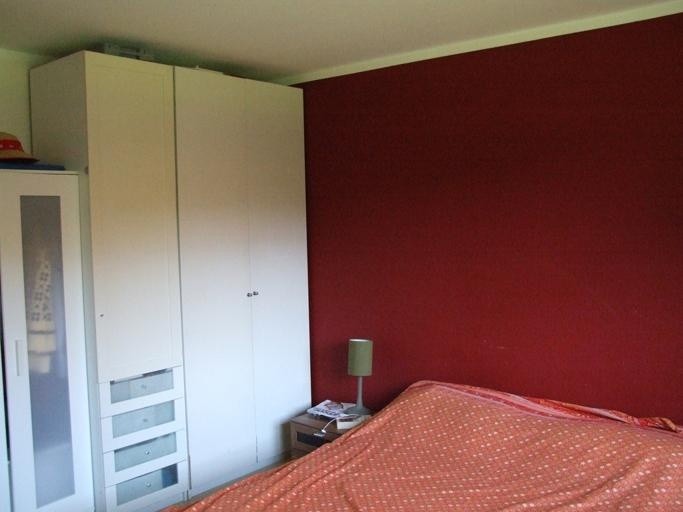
[151, 378, 682, 511]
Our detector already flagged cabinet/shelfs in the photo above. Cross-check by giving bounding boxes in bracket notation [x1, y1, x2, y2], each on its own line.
[28, 51, 189, 511]
[0, 170, 96, 511]
[173, 64, 313, 492]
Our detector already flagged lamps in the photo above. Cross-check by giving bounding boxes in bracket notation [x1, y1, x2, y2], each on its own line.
[346, 338, 373, 418]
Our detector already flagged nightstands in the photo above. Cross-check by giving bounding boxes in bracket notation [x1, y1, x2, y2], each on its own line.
[290, 413, 353, 463]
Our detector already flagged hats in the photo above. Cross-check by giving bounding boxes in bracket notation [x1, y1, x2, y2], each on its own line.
[0, 132, 40, 162]
